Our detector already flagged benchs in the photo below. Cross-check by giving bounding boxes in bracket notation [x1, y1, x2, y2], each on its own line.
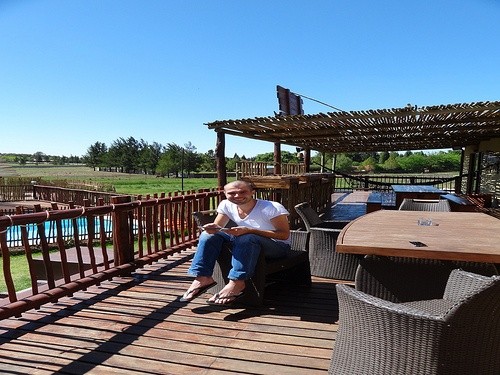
[440, 194, 477, 212]
[366, 193, 382, 214]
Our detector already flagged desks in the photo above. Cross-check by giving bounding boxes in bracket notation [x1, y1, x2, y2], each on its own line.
[337, 210, 500, 290]
[391, 185, 449, 210]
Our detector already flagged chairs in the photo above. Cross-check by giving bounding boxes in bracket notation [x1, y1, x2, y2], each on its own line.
[398, 198, 451, 212]
[294, 201, 364, 281]
[328, 269, 500, 375]
[192, 210, 312, 307]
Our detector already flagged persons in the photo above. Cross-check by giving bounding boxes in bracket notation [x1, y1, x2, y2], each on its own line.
[179, 181, 291, 306]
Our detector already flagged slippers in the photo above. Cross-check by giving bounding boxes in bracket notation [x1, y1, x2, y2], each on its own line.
[205, 291, 246, 305]
[179, 281, 216, 302]
